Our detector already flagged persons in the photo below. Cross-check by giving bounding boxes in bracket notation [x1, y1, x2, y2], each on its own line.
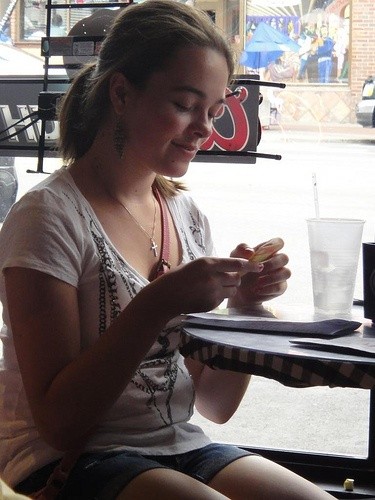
[1, 0, 340, 500]
[297, 24, 338, 83]
[265, 59, 290, 125]
[24, 3, 94, 40]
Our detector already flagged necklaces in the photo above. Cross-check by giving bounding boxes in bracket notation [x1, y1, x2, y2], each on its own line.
[82, 155, 160, 256]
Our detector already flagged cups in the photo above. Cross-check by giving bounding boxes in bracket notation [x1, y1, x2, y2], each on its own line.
[360, 239, 374, 322]
[306, 214, 365, 314]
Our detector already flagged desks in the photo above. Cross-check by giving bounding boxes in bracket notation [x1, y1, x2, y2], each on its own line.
[180, 301, 374, 392]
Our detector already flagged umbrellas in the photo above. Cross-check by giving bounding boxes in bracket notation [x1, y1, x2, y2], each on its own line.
[243, 21, 298, 67]
